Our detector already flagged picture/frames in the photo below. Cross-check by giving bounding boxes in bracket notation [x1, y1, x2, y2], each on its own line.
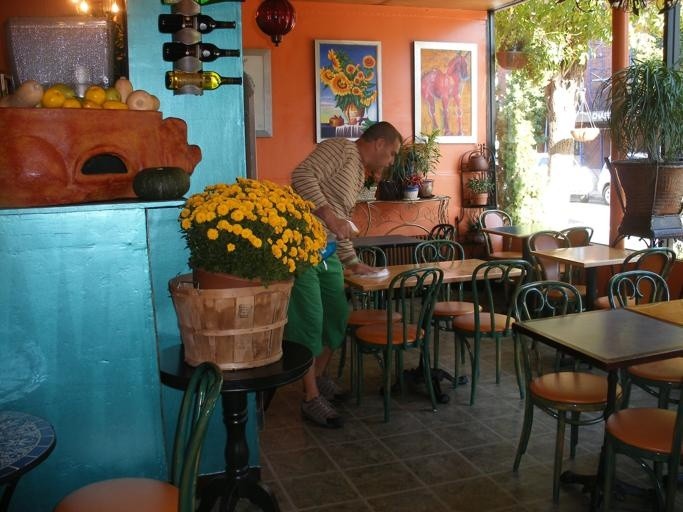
[412, 40, 478, 144]
[241, 47, 273, 138]
[314, 39, 383, 144]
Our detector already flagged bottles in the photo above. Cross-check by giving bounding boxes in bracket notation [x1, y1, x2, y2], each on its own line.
[158, 0, 242, 91]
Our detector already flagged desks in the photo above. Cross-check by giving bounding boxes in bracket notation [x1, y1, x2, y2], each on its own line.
[513, 307, 683, 509]
[629, 295, 683, 327]
[157, 338, 314, 511]
[349, 194, 449, 234]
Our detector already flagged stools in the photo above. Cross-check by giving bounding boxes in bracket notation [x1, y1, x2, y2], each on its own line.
[612, 214, 683, 256]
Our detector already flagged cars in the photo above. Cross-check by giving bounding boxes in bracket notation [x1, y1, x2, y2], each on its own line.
[597, 151, 648, 207]
[528, 151, 597, 201]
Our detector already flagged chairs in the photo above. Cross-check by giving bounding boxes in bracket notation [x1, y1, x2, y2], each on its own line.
[511, 281, 622, 506]
[54, 361, 225, 511]
[337, 210, 676, 424]
[608, 271, 680, 417]
[598, 379, 682, 508]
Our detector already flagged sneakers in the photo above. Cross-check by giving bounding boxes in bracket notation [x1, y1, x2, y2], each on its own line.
[302, 395, 344, 428]
[316, 376, 351, 401]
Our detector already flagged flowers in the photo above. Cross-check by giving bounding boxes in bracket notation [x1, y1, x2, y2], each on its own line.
[178, 174, 329, 286]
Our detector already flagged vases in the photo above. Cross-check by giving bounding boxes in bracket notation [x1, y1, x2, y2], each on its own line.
[188, 262, 290, 290]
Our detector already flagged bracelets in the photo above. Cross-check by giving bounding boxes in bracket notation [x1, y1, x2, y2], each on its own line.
[345, 257, 358, 266]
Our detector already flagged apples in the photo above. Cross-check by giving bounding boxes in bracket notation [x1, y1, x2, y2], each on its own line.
[34, 84, 128, 109]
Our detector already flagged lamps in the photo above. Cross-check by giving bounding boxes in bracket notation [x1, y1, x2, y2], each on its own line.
[255, 0, 297, 48]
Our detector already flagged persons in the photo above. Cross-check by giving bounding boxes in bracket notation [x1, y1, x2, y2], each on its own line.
[283, 121, 402, 430]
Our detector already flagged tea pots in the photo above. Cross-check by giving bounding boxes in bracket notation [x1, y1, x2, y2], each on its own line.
[468, 151, 492, 171]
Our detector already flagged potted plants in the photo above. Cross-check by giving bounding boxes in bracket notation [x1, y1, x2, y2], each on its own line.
[591, 56, 683, 217]
[356, 127, 444, 202]
[495, 30, 557, 94]
[466, 176, 496, 206]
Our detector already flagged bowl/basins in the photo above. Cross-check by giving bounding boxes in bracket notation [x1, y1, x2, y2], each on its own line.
[329, 118, 344, 128]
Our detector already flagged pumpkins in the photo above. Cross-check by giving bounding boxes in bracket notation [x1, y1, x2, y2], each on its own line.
[115, 77, 160, 110]
[0, 80, 45, 108]
[133, 166, 190, 200]
